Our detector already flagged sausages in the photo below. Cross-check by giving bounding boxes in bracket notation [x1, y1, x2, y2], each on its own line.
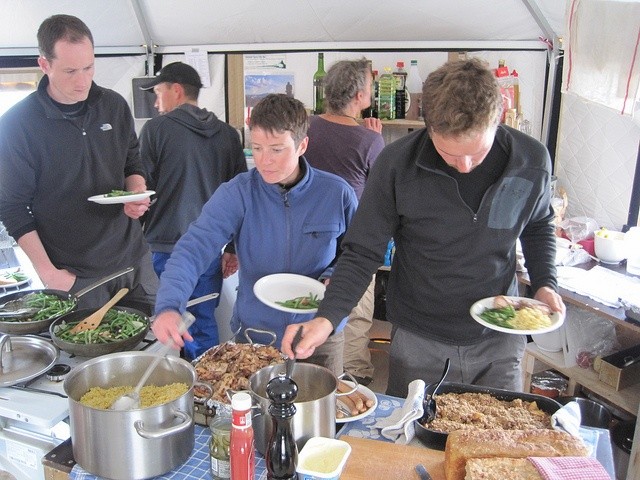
[337, 380, 363, 409]
[337, 390, 358, 416]
[352, 388, 373, 407]
[360, 404, 367, 414]
[336, 413, 344, 418]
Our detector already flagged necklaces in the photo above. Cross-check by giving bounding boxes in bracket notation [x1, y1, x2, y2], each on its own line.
[341, 113, 356, 121]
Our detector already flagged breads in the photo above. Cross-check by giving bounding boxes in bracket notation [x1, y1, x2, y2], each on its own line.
[445, 430, 591, 480]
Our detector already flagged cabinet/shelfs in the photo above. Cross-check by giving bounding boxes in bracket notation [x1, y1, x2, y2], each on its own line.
[516, 260, 639, 479]
[0, 318, 184, 480]
[357, 118, 426, 133]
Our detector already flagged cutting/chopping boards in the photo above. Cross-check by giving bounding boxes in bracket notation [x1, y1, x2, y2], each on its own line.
[338, 435, 446, 480]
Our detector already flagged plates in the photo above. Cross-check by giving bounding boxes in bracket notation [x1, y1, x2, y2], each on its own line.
[253, 272, 327, 314]
[470, 295, 565, 335]
[335, 380, 379, 423]
[87, 190, 156, 205]
[0, 272, 32, 288]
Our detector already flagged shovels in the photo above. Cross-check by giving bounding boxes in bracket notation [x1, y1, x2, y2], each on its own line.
[70, 288, 129, 336]
[109, 311, 196, 412]
[419, 357, 450, 425]
[0, 305, 43, 322]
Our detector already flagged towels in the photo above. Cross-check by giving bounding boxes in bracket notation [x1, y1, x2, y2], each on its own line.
[366, 378, 425, 447]
[550, 398, 600, 458]
[528, 455, 610, 479]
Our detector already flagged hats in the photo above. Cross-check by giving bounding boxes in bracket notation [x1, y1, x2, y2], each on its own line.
[139, 63, 203, 93]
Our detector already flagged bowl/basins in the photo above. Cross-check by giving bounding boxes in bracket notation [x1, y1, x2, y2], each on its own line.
[531, 331, 563, 353]
[594, 230, 627, 265]
[550, 397, 612, 432]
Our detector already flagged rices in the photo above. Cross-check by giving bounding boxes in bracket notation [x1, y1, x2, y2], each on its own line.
[464, 456, 611, 480]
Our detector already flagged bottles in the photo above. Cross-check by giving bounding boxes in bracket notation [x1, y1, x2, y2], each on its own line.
[265, 373, 299, 480]
[407, 60, 423, 120]
[360, 60, 373, 119]
[378, 67, 395, 120]
[373, 70, 379, 119]
[209, 415, 229, 480]
[393, 62, 407, 119]
[229, 393, 255, 480]
[312, 53, 328, 114]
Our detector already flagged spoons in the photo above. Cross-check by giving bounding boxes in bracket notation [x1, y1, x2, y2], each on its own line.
[423, 357, 451, 424]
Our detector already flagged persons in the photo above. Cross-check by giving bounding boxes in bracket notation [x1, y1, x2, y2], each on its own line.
[138, 62, 247, 357]
[281, 60, 566, 400]
[301, 61, 388, 388]
[151, 93, 358, 380]
[1, 14, 162, 318]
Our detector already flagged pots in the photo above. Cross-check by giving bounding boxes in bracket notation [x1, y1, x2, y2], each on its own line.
[48, 292, 220, 357]
[611, 421, 636, 480]
[0, 266, 135, 334]
[225, 362, 359, 456]
